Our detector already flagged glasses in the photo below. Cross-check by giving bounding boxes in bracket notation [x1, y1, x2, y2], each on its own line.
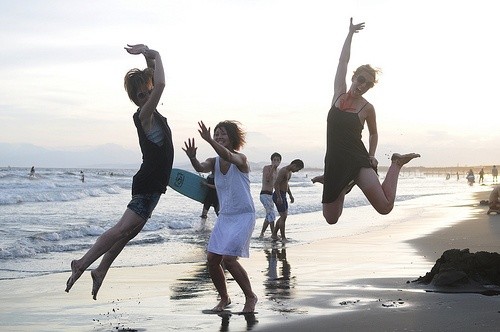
[355, 75, 374, 88]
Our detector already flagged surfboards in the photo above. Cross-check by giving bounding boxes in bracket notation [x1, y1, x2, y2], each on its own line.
[163, 169, 223, 204]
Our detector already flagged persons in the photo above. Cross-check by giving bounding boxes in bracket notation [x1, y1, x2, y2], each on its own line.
[487, 184, 500, 214]
[260, 153, 281, 237]
[271, 159, 304, 243]
[29, 165, 85, 185]
[65, 44, 174, 300]
[311, 17, 421, 225]
[445, 166, 499, 185]
[182, 120, 259, 313]
[199, 170, 220, 220]
